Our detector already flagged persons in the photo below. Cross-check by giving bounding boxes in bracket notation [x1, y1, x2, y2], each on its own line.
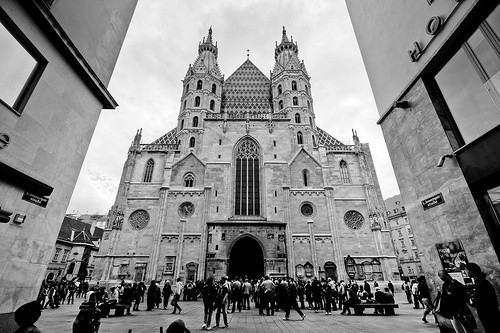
[214, 275, 230, 327]
[411, 279, 425, 309]
[162, 278, 172, 311]
[384, 286, 396, 315]
[418, 275, 439, 324]
[435, 270, 477, 333]
[12, 299, 43, 333]
[71, 300, 101, 333]
[439, 243, 469, 273]
[283, 276, 306, 320]
[171, 276, 183, 314]
[374, 281, 378, 286]
[183, 271, 363, 314]
[202, 275, 217, 331]
[464, 262, 500, 333]
[340, 288, 361, 315]
[35, 274, 161, 315]
[373, 286, 386, 314]
[364, 280, 371, 300]
[402, 276, 414, 302]
[388, 280, 394, 297]
[358, 285, 368, 301]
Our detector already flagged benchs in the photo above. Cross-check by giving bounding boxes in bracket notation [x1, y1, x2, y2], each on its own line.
[352, 303, 399, 316]
[79, 304, 130, 317]
